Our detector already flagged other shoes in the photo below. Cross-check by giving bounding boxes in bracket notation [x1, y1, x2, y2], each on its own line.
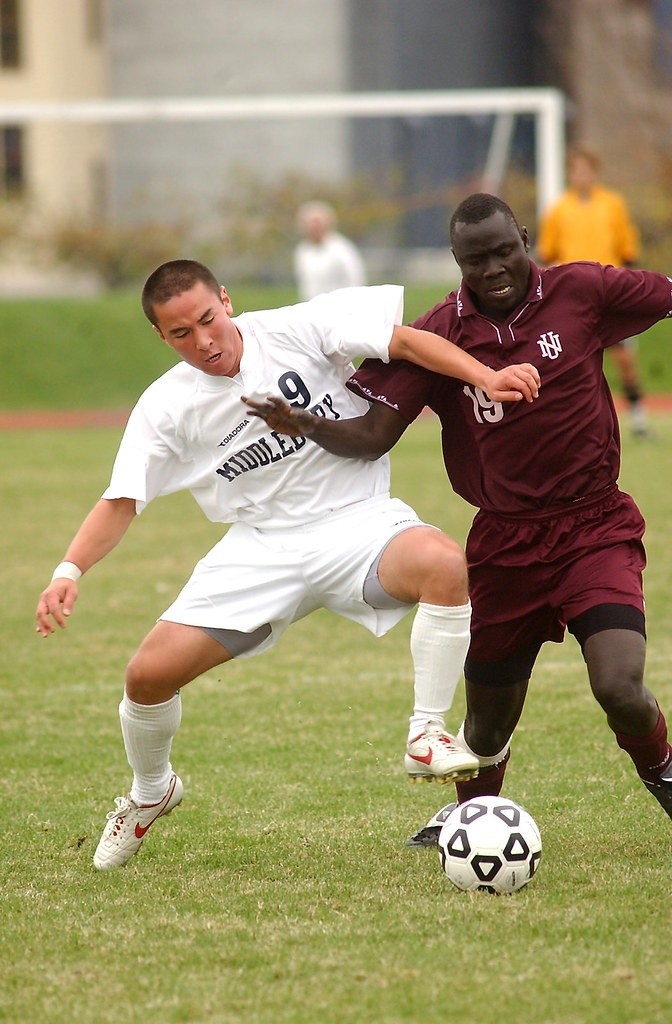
[405, 800, 460, 850]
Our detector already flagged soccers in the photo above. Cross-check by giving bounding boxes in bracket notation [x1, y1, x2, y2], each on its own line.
[435, 795, 543, 895]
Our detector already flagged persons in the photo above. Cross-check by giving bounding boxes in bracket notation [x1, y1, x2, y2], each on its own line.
[532, 137, 649, 439]
[36, 260, 541, 871]
[292, 200, 369, 306]
[239, 190, 672, 847]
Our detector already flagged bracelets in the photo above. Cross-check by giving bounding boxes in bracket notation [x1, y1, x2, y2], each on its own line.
[51, 561, 84, 583]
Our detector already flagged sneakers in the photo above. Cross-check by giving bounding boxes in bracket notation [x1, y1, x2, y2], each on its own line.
[93, 771, 183, 871]
[404, 720, 480, 785]
